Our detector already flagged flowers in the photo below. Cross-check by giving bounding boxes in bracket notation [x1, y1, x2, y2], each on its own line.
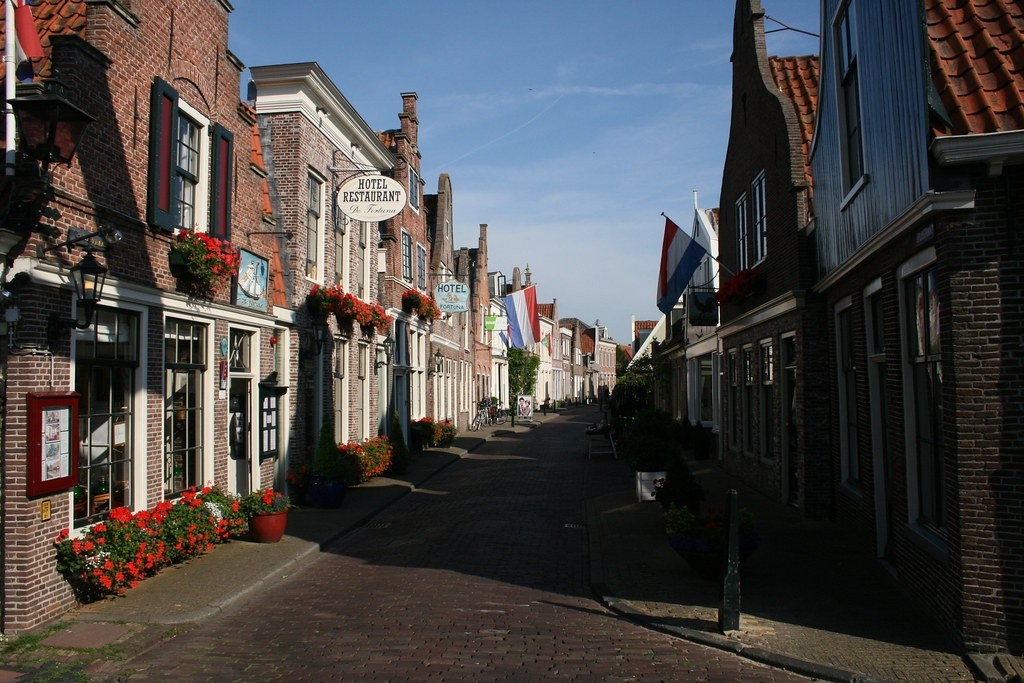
[665, 501, 763, 546]
[240, 488, 302, 517]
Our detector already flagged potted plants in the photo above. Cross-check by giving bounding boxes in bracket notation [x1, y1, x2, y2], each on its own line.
[386, 409, 413, 474]
[303, 412, 347, 507]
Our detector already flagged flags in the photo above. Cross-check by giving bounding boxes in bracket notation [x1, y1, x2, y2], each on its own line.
[656, 217, 708, 315]
[499, 322, 514, 350]
[541, 332, 553, 356]
[501, 285, 541, 349]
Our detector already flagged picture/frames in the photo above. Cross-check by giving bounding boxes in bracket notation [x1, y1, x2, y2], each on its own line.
[113, 421, 126, 446]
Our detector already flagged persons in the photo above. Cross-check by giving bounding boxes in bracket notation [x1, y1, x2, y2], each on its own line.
[518, 397, 525, 416]
[520, 400, 532, 417]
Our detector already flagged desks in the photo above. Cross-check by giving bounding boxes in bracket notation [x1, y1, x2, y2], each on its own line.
[586, 426, 618, 460]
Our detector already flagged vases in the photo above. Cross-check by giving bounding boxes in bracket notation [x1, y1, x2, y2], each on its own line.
[668, 539, 754, 572]
[251, 510, 288, 543]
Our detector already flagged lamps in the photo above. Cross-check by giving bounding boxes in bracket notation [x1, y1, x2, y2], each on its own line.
[45, 238, 109, 339]
[5, 64, 97, 268]
[36, 221, 126, 261]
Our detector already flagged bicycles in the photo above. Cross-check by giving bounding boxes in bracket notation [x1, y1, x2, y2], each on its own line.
[469, 399, 508, 432]
